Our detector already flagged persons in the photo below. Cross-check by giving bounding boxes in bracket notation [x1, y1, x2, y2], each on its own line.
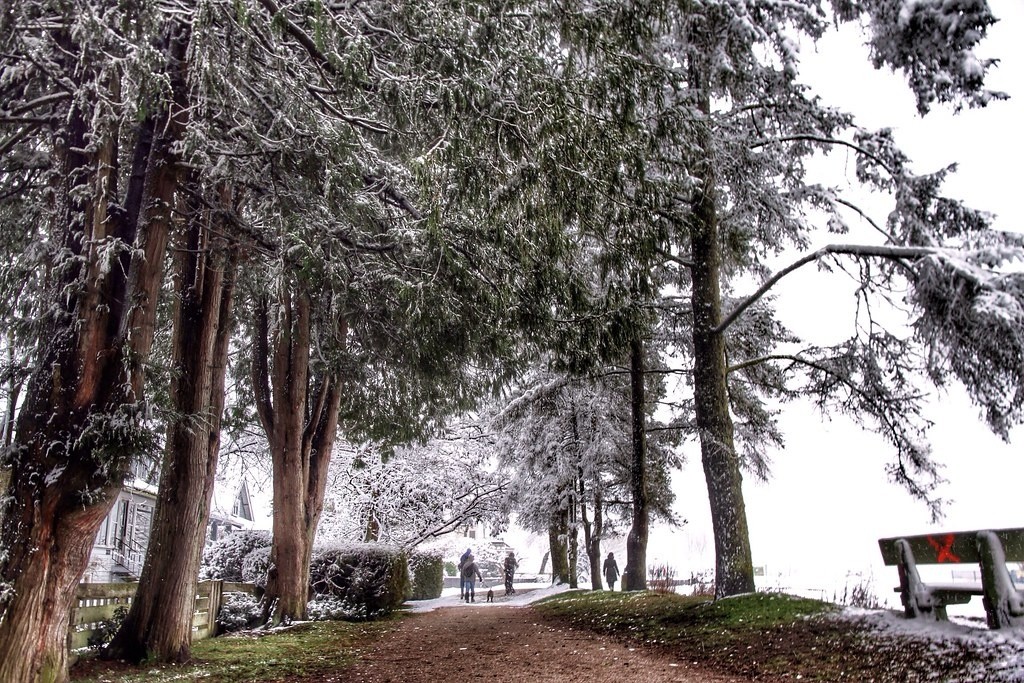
[504, 553, 519, 596]
[602, 552, 620, 592]
[458, 548, 483, 603]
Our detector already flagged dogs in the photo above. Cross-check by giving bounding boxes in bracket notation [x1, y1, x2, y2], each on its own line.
[487, 588, 494, 602]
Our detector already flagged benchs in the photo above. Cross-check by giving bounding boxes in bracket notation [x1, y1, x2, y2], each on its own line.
[877, 527, 1024, 630]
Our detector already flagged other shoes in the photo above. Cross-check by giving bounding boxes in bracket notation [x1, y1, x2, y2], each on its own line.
[461, 594, 464, 599]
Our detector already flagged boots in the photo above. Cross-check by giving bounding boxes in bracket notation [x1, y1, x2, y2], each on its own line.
[464, 592, 469, 603]
[471, 592, 475, 601]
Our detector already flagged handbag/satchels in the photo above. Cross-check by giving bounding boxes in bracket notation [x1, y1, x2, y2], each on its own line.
[464, 563, 474, 577]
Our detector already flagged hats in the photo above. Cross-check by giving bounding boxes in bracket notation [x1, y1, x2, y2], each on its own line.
[608, 553, 614, 558]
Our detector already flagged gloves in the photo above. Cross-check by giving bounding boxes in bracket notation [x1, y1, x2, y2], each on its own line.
[480, 577, 482, 582]
